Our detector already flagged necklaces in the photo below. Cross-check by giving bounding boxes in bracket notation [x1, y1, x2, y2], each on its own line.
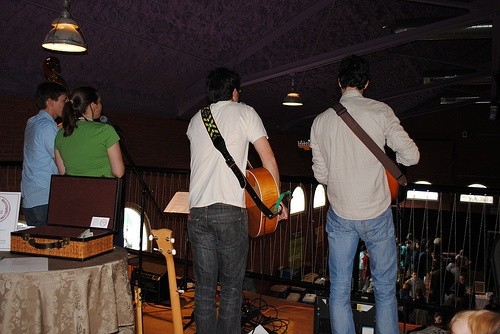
[78, 116, 94, 121]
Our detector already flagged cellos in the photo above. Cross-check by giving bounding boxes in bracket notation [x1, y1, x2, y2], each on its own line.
[42, 56, 68, 94]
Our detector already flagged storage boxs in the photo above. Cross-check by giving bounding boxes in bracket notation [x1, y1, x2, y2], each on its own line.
[9, 174, 123, 262]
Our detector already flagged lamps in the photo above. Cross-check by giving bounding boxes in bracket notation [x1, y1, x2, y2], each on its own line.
[42, 0, 87, 54]
[282, 75, 303, 108]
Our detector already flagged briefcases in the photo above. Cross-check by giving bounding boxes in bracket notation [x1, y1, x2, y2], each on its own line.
[11, 174, 119, 262]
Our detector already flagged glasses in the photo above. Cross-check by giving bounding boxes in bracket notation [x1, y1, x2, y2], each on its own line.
[232, 87, 243, 93]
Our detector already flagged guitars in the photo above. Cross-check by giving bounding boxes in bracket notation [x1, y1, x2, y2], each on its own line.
[297, 139, 407, 204]
[149, 228, 184, 334]
[246, 168, 279, 238]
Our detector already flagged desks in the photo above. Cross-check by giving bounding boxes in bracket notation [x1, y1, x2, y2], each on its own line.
[0, 248, 136, 334]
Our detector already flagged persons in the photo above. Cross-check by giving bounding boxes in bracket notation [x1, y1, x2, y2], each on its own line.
[358, 246, 368, 282]
[310, 54, 420, 334]
[394, 233, 500, 334]
[54, 86, 125, 177]
[20, 81, 66, 226]
[186, 67, 288, 334]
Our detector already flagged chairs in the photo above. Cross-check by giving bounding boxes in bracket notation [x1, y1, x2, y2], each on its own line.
[123, 201, 153, 272]
